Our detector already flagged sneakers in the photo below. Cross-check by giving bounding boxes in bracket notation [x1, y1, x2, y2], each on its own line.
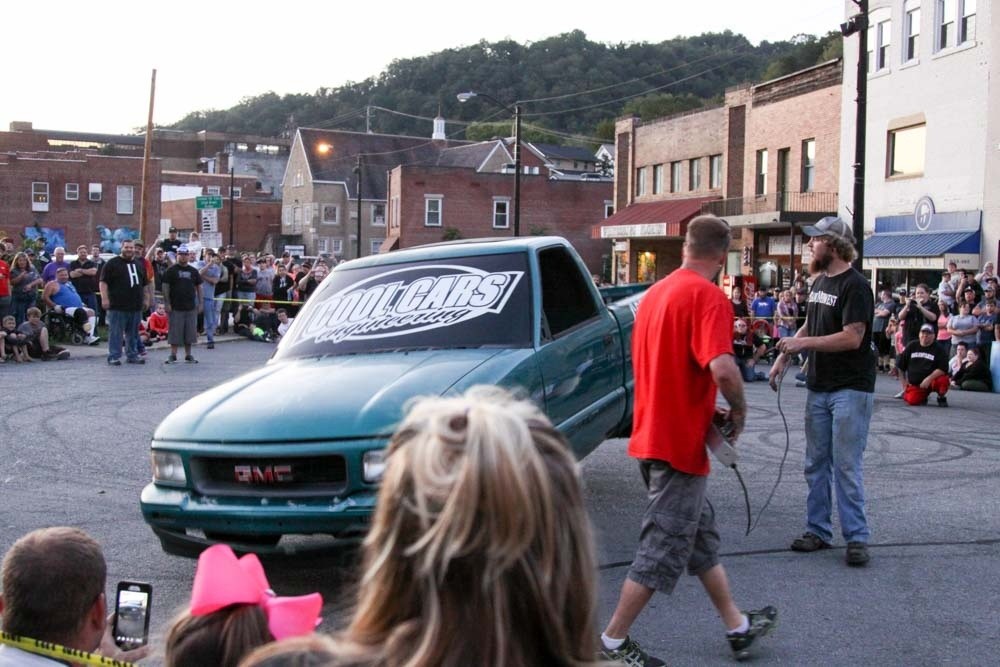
[790, 531, 831, 552]
[726, 605, 781, 660]
[594, 633, 667, 667]
[847, 541, 871, 565]
[80, 328, 227, 365]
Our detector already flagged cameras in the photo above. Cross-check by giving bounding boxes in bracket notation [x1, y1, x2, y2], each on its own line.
[315, 270, 324, 277]
[911, 299, 919, 305]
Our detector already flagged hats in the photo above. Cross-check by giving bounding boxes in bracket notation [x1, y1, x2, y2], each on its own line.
[177, 247, 189, 255]
[775, 287, 781, 292]
[25, 248, 34, 256]
[900, 289, 906, 295]
[921, 324, 934, 333]
[797, 288, 806, 295]
[986, 277, 998, 284]
[133, 239, 144, 247]
[218, 247, 225, 251]
[984, 287, 993, 292]
[169, 227, 177, 233]
[800, 217, 852, 242]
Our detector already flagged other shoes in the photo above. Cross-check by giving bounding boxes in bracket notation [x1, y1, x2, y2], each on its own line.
[760, 371, 769, 380]
[0, 352, 57, 363]
[795, 379, 806, 386]
[894, 391, 904, 399]
[937, 396, 948, 407]
[878, 364, 898, 376]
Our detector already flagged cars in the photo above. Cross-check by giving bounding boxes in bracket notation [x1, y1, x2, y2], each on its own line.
[50, 252, 117, 264]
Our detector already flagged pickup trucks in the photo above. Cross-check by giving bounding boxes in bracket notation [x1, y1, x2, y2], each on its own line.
[137, 234, 662, 559]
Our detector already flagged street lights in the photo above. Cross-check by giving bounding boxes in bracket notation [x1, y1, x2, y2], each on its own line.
[457, 92, 521, 236]
[200, 154, 235, 246]
[314, 137, 362, 259]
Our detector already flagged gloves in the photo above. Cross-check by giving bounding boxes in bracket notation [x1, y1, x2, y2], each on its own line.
[54, 305, 62, 314]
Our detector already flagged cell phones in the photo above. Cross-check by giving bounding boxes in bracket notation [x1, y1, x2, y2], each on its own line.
[111, 580, 154, 651]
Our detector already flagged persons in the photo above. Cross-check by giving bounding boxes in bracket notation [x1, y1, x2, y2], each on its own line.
[597, 214, 777, 667]
[728, 261, 1000, 407]
[0, 227, 346, 366]
[0, 526, 151, 667]
[768, 217, 877, 566]
[165, 385, 596, 666]
